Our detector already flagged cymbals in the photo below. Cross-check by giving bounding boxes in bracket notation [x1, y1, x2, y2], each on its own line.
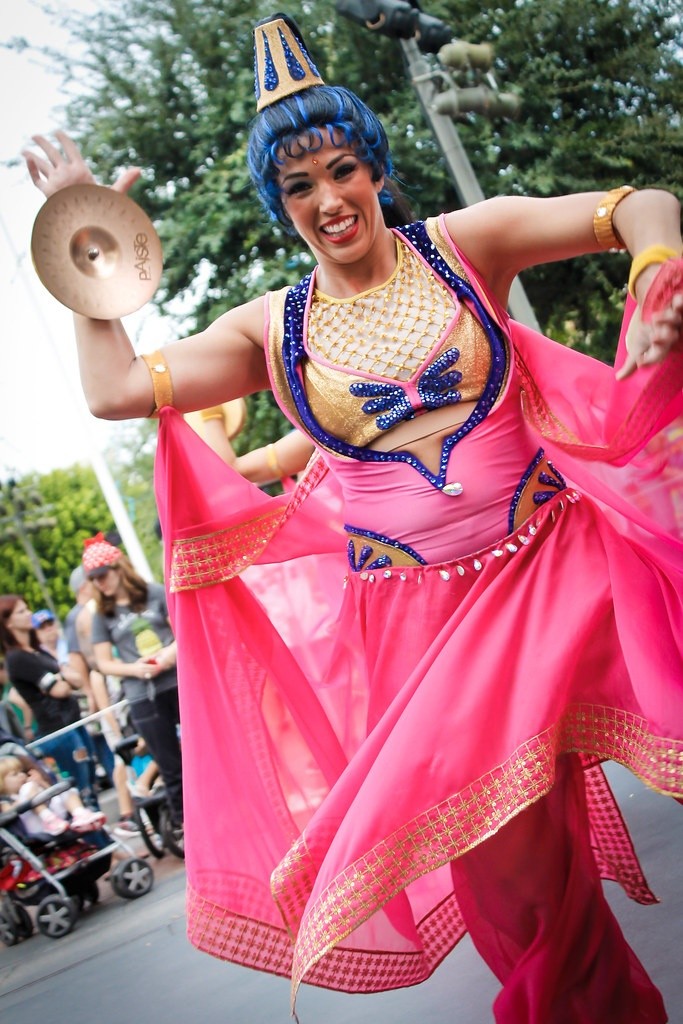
[30, 183, 163, 320]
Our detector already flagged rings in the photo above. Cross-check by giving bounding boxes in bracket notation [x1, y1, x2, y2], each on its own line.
[145, 673, 151, 679]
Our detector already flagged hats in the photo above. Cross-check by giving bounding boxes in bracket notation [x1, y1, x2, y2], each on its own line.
[69, 566, 88, 593]
[31, 609, 55, 629]
[82, 542, 123, 575]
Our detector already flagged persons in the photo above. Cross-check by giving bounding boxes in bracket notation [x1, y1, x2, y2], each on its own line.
[20, 13, 682, 1023]
[0, 404, 316, 881]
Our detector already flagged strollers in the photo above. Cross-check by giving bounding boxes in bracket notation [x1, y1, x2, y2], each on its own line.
[0, 728, 153, 946]
[113, 702, 185, 860]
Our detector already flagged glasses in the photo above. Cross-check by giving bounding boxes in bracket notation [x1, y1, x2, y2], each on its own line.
[88, 574, 106, 582]
[38, 620, 54, 629]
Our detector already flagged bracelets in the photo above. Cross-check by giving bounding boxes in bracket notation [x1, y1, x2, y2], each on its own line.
[628, 244, 680, 301]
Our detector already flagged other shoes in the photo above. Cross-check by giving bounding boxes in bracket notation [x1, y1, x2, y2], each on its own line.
[42, 816, 69, 836]
[119, 815, 140, 832]
[70, 811, 106, 832]
